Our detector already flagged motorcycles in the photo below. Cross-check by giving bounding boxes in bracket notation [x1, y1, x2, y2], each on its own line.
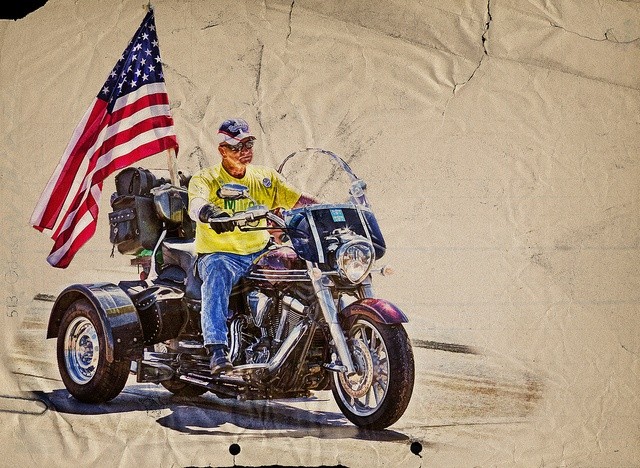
[45, 146, 415, 431]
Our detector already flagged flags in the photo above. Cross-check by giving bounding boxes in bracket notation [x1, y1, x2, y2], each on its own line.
[30, 11, 179, 269]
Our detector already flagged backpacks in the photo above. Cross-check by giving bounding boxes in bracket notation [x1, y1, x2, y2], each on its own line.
[107, 166, 160, 255]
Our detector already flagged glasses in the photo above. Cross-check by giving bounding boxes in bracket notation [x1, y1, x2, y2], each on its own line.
[225, 139, 253, 150]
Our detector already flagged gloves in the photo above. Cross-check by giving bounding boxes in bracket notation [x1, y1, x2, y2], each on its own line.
[199, 205, 235, 234]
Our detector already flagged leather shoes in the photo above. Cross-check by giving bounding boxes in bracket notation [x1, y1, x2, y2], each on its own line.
[204, 342, 233, 374]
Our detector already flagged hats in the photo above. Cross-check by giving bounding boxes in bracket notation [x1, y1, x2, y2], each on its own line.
[216, 118, 256, 146]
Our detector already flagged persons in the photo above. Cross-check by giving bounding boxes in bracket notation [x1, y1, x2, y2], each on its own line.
[188, 119, 326, 373]
